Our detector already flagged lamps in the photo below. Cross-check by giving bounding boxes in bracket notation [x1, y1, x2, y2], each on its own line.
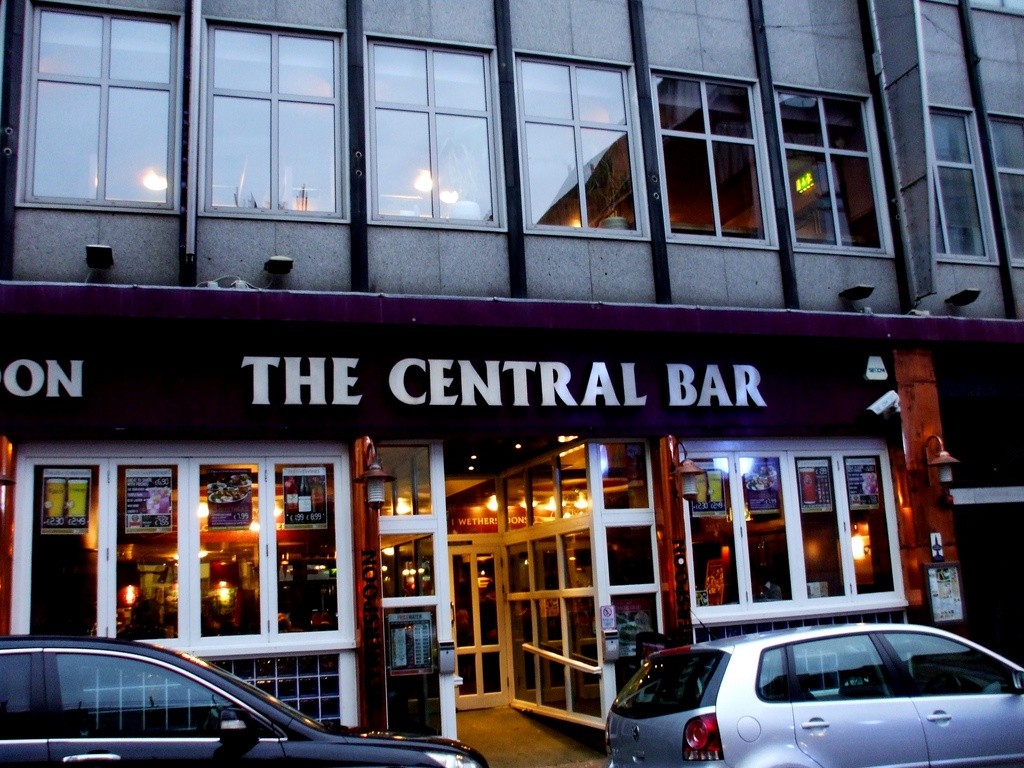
[85, 242, 114, 272]
[667, 440, 705, 501]
[924, 435, 961, 489]
[945, 287, 981, 307]
[263, 257, 295, 274]
[838, 285, 874, 302]
[355, 442, 399, 510]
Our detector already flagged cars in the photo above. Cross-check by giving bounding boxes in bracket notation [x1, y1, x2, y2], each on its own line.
[598, 614, 1023, 768]
[0, 631, 492, 768]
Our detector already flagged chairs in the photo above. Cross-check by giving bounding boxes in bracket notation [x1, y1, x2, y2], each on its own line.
[784, 666, 816, 702]
[837, 656, 880, 697]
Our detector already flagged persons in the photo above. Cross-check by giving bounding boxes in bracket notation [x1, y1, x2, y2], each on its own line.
[149, 586, 170, 627]
[480, 581, 538, 645]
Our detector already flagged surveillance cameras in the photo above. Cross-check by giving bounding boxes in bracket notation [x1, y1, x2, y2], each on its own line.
[867, 390, 901, 417]
